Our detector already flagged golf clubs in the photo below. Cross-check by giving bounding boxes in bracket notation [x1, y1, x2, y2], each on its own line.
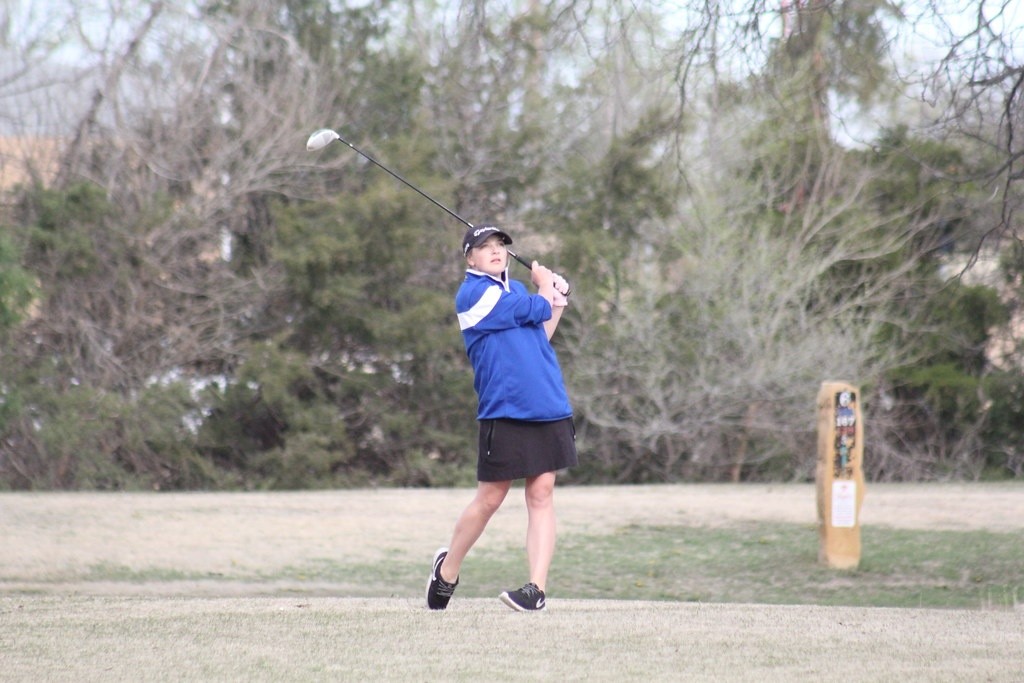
[305, 127, 572, 298]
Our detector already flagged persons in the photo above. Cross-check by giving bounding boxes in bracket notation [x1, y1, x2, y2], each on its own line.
[426, 224, 577, 612]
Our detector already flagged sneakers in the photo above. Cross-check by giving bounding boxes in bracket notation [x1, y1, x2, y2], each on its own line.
[427, 549, 459, 610]
[499, 583, 546, 612]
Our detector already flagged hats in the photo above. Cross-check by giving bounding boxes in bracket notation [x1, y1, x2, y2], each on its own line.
[463, 224, 512, 257]
